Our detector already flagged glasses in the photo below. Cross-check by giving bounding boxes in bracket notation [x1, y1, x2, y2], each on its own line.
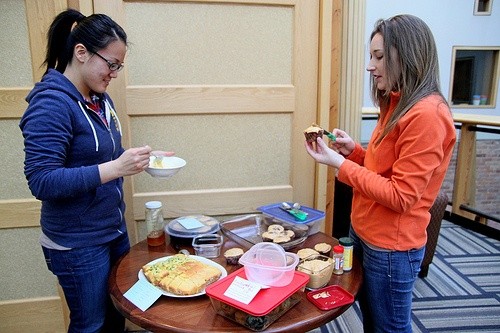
[87, 49, 124, 72]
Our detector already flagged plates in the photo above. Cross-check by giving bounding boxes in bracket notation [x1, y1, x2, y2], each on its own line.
[137, 253, 228, 299]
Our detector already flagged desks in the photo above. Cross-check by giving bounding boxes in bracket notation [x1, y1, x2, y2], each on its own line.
[110, 220, 364, 333]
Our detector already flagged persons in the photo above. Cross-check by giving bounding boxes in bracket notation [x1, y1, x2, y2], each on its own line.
[17, 8, 175, 333]
[301, 14, 457, 332]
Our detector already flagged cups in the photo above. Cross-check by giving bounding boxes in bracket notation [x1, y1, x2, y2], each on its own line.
[314, 242, 332, 261]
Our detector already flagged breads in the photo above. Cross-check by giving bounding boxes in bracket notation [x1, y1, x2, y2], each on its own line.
[142, 254, 222, 296]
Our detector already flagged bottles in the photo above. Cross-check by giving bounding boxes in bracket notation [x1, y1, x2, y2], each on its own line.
[333, 246, 344, 275]
[472, 95, 488, 105]
[338, 236, 354, 271]
[144, 200, 164, 247]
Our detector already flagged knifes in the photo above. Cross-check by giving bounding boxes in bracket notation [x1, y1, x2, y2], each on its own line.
[311, 122, 336, 141]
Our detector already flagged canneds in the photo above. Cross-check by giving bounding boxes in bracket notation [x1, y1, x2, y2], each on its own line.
[145, 201, 166, 247]
[338, 237, 353, 271]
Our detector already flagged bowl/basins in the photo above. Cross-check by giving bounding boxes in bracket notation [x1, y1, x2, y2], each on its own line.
[192, 232, 224, 258]
[165, 214, 220, 246]
[204, 267, 311, 329]
[238, 241, 300, 288]
[143, 156, 186, 177]
[255, 201, 325, 237]
[294, 252, 334, 292]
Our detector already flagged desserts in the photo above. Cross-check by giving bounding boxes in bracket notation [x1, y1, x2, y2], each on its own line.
[224, 248, 244, 266]
[261, 224, 295, 245]
[303, 126, 323, 143]
[296, 243, 332, 261]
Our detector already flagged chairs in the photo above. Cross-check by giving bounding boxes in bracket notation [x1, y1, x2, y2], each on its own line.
[334, 168, 448, 278]
